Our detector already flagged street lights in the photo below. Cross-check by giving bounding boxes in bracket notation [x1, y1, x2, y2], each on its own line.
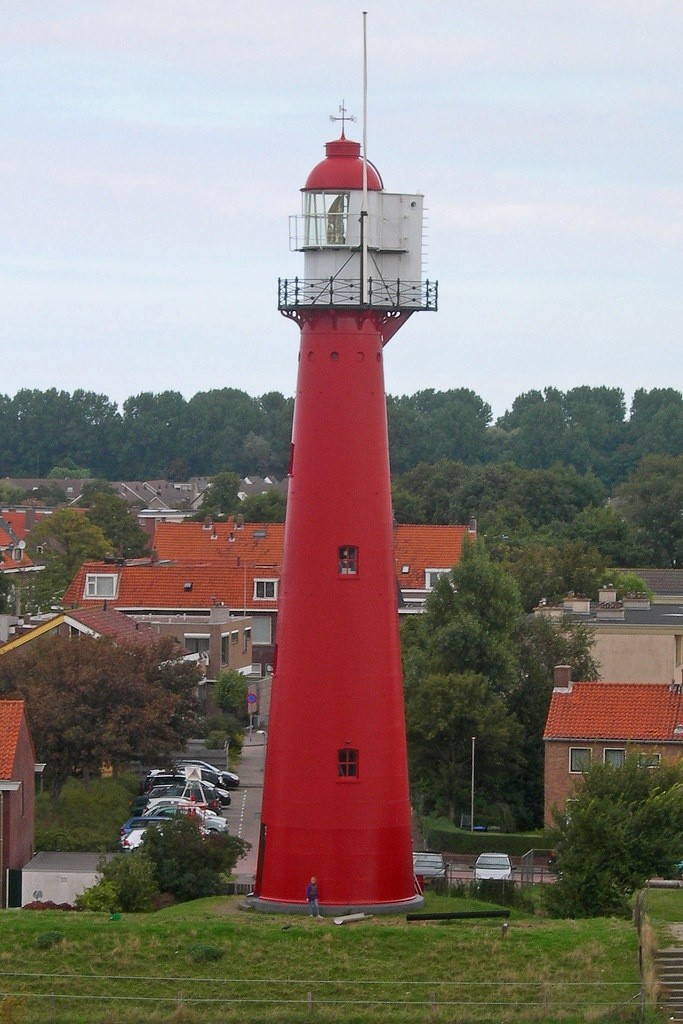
[471, 736, 476, 833]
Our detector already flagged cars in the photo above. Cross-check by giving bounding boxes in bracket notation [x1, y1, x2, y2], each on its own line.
[121, 830, 162, 855]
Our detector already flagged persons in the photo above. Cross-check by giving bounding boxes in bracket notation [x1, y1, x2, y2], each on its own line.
[306, 877, 323, 918]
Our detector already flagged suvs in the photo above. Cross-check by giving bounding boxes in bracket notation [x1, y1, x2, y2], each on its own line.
[119, 818, 210, 840]
[142, 805, 231, 833]
[131, 759, 241, 817]
[142, 798, 216, 817]
[469, 852, 517, 882]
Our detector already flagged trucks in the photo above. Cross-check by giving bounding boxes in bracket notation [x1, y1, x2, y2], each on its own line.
[413, 852, 450, 894]
